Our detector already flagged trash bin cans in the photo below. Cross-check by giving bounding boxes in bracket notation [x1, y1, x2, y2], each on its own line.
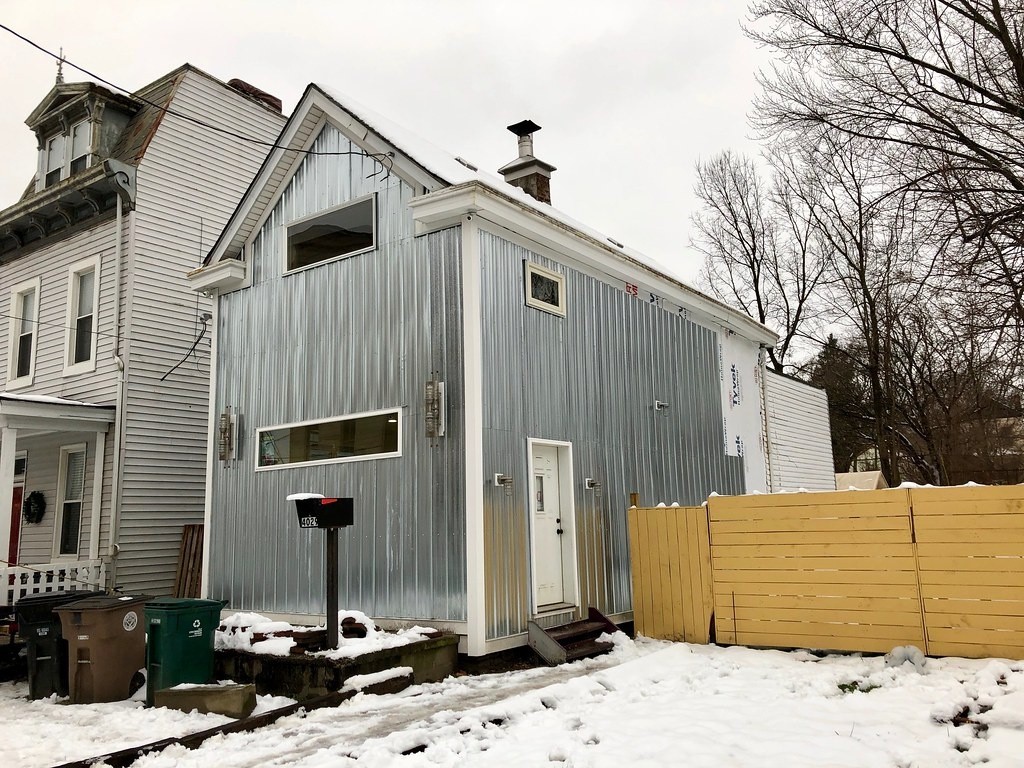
[51, 594, 150, 705]
[142, 595, 229, 708]
[14, 590, 107, 701]
